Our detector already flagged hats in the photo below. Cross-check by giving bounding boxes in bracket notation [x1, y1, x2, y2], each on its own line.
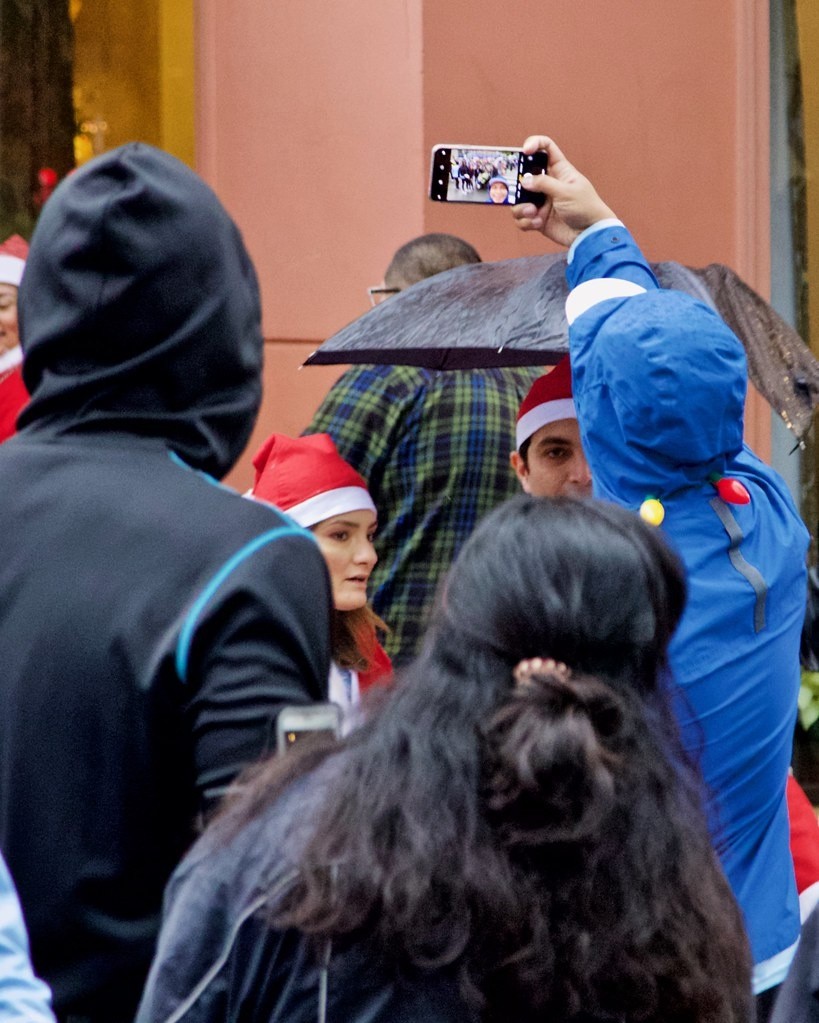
[0, 234, 29, 287]
[252, 433, 376, 533]
[516, 359, 579, 454]
[564, 276, 645, 326]
[489, 178, 507, 186]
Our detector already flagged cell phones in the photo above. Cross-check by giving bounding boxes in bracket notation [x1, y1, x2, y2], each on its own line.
[276, 703, 342, 759]
[428, 143, 548, 207]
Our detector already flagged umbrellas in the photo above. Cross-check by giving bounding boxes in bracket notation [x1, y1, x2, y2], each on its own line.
[297, 251, 817, 454]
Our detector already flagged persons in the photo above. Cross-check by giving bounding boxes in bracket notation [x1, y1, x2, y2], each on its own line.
[447, 150, 517, 204]
[0, 132, 819, 1023]
[135, 493, 753, 1022]
